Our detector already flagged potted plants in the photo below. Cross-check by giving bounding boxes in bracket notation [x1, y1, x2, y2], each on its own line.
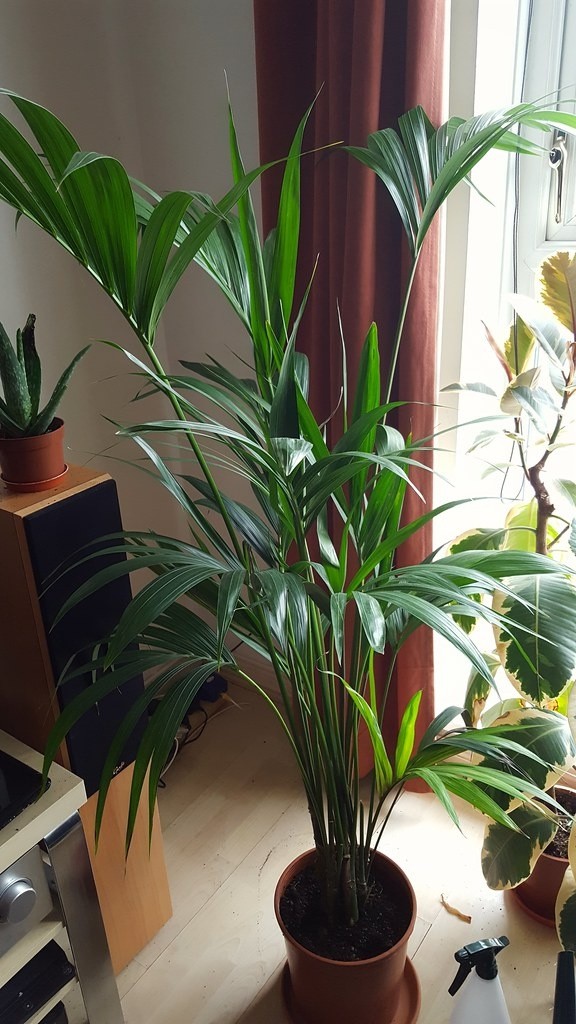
[0, 85, 576, 1024]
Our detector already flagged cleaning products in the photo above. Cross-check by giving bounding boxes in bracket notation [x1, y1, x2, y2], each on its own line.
[448, 934, 511, 1024]
